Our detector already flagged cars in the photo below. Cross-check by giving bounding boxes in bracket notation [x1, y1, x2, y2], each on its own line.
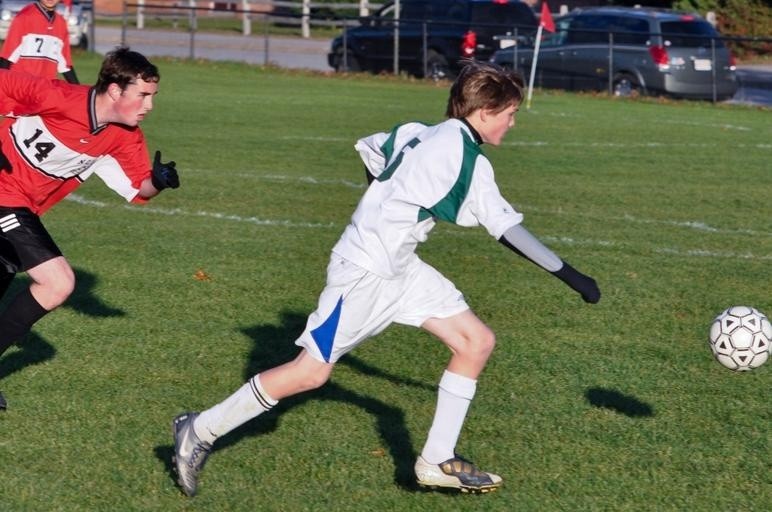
[487, 7, 740, 101]
[0, 0, 94, 51]
[324, 0, 543, 85]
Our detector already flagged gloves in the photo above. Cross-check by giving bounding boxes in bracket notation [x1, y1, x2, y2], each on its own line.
[152, 150, 179, 190]
[557, 264, 601, 304]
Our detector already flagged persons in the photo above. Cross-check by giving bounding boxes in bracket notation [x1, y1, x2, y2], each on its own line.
[172, 63, 600, 498]
[1, 0, 80, 85]
[55, 1, 88, 52]
[1, 48, 179, 412]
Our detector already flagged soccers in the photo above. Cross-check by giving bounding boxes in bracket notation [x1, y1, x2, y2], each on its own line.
[709, 306, 772, 372]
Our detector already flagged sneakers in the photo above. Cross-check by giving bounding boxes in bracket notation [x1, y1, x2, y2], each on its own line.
[173, 411, 210, 497]
[412, 455, 503, 494]
